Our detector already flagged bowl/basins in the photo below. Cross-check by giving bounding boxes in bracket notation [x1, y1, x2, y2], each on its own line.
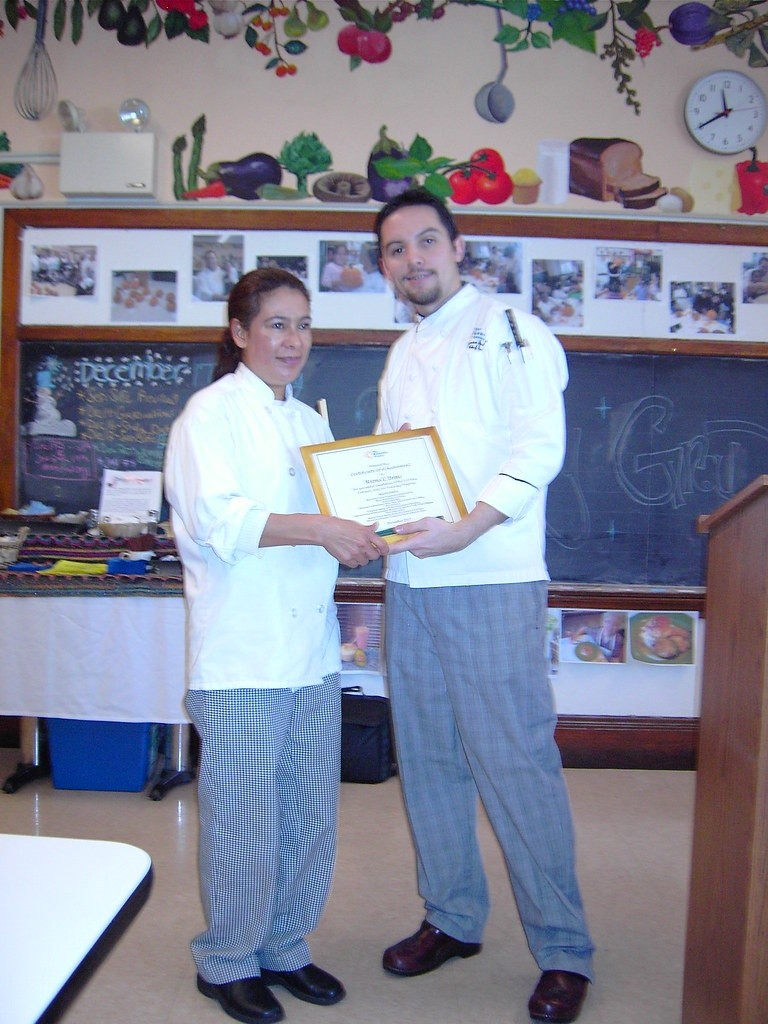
[99, 523, 146, 537]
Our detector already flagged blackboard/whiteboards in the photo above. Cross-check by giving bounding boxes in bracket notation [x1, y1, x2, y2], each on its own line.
[0, 331, 768, 597]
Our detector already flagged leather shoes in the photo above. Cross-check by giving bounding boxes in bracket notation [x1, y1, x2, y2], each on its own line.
[383, 919, 481, 976]
[260, 963, 346, 1006]
[528, 970, 589, 1024]
[197, 970, 285, 1024]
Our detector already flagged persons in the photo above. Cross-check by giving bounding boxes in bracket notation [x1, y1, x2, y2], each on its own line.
[372, 189, 598, 1023]
[532, 261, 582, 323]
[257, 257, 307, 280]
[595, 254, 661, 301]
[394, 242, 522, 323]
[670, 281, 734, 334]
[742, 257, 768, 304]
[321, 244, 387, 292]
[196, 248, 240, 301]
[571, 612, 625, 662]
[29, 246, 97, 296]
[164, 268, 388, 1024]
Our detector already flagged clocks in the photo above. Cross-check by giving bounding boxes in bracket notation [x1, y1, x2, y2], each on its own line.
[684, 69, 768, 156]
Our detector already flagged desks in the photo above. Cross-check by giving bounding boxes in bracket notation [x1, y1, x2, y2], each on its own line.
[0, 596, 194, 802]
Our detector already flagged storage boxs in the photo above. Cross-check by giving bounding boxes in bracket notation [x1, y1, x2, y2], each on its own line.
[45, 718, 152, 792]
[149, 726, 160, 778]
